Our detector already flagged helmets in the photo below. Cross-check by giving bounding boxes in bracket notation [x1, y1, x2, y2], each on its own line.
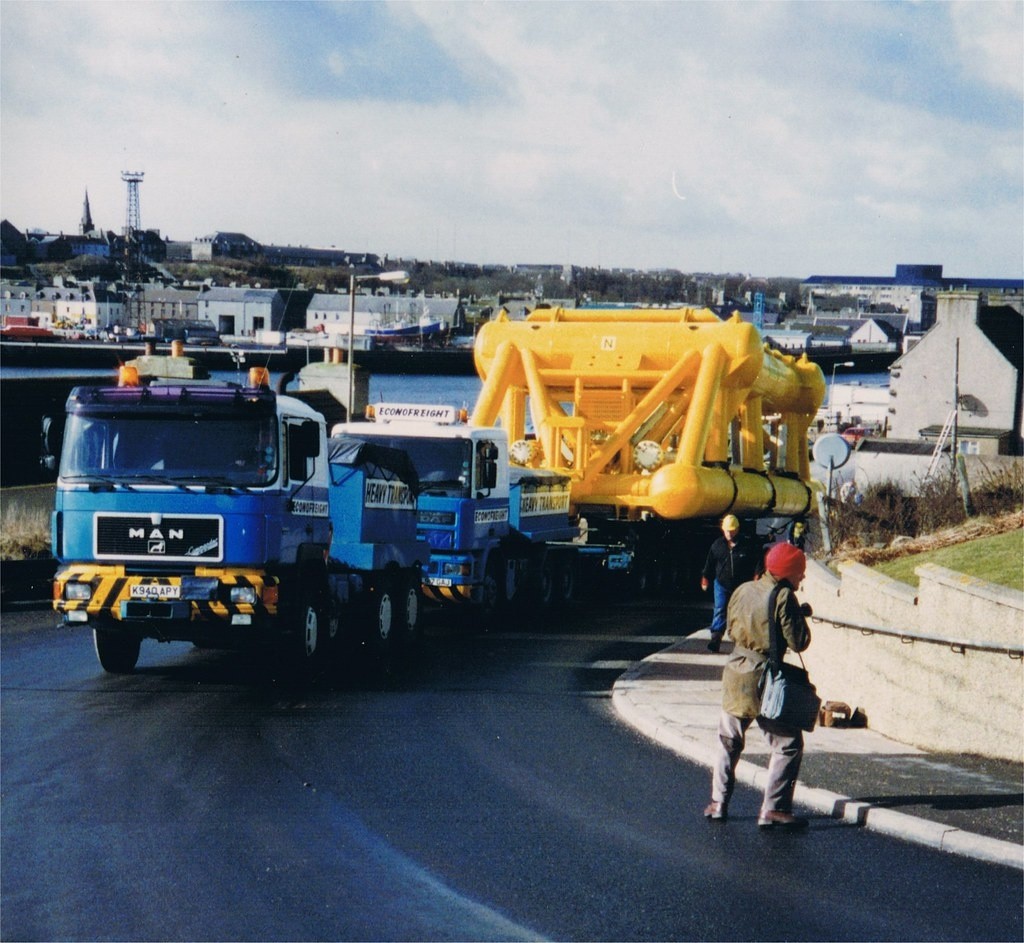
[722, 515, 739, 531]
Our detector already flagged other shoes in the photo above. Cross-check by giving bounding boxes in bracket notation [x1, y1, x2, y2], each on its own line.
[703, 801, 727, 820]
[758, 802, 809, 828]
[706, 632, 721, 653]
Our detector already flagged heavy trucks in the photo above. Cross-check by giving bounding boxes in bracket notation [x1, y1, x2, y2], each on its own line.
[329, 402, 578, 633]
[46, 334, 423, 684]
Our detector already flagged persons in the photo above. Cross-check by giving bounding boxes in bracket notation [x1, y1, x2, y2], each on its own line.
[704, 541, 813, 828]
[701, 515, 765, 653]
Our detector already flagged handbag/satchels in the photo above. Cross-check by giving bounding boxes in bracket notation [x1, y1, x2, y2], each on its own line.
[756, 658, 820, 732]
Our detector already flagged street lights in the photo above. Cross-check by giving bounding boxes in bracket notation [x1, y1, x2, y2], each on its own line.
[345, 268, 413, 420]
[826, 361, 854, 434]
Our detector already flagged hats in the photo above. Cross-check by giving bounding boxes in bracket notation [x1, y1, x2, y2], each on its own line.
[766, 543, 806, 577]
